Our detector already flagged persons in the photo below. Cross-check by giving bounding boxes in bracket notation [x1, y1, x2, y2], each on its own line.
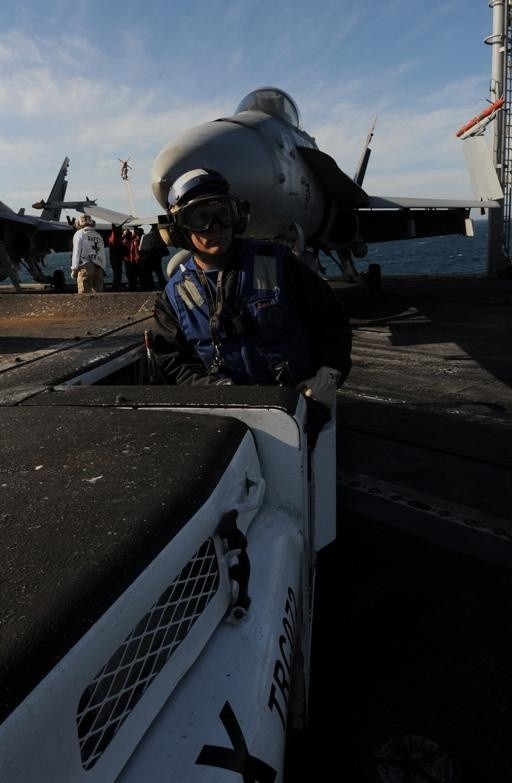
[70, 214, 109, 293]
[144, 166, 355, 396]
[105, 222, 170, 293]
[336, 201, 361, 283]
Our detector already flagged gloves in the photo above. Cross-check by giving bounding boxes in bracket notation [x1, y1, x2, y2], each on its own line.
[295, 366, 343, 410]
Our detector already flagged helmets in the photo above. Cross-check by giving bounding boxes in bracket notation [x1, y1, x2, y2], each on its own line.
[166, 168, 239, 212]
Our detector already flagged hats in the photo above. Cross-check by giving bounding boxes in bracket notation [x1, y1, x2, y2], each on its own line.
[78, 215, 95, 226]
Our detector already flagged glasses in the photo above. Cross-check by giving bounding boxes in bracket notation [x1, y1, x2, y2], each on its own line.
[177, 199, 240, 233]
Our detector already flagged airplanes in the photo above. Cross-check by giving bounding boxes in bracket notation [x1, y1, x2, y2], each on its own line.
[118, 156, 132, 180]
[32, 196, 155, 227]
[31, 85, 505, 302]
[0, 157, 122, 295]
